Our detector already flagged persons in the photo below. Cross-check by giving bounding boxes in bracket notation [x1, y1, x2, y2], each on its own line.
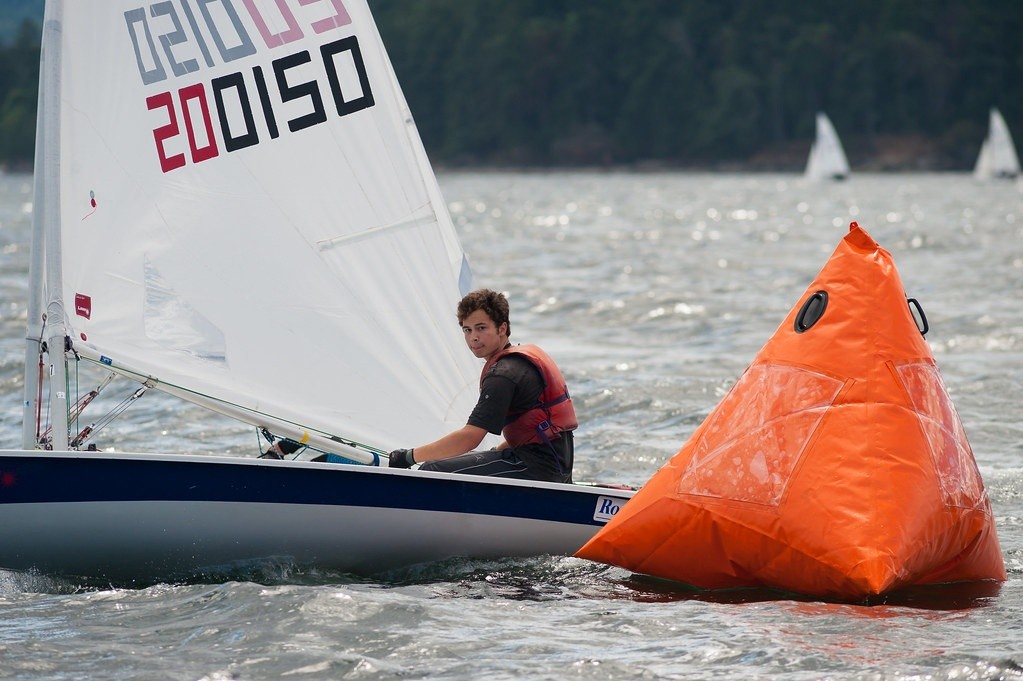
[389, 290, 579, 484]
[266, 436, 379, 467]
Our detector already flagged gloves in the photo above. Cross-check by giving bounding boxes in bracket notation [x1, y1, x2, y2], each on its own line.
[389, 448, 417, 468]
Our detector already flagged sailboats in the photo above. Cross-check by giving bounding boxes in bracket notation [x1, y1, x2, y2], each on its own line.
[0, 0, 648, 597]
[799, 109, 853, 187]
[968, 105, 1023, 186]
